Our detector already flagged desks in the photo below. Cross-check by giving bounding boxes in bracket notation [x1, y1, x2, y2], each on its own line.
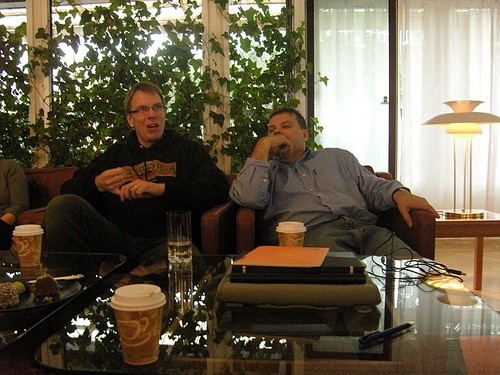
[434, 208, 500, 290]
[0, 247, 500, 375]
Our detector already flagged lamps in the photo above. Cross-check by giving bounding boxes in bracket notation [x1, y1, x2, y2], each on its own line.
[421, 100, 500, 218]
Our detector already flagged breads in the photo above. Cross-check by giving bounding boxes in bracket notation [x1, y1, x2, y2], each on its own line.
[0, 281, 20, 308]
[33, 274, 60, 297]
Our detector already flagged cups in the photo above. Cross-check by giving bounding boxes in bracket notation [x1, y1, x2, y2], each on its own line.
[12, 224, 44, 267]
[110, 284, 167, 366]
[168, 261, 194, 315]
[166, 211, 193, 263]
[275, 221, 307, 247]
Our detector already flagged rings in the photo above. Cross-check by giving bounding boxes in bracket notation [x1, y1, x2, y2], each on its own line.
[134, 186, 137, 188]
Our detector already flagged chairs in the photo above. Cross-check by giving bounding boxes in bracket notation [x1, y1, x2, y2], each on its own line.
[32, 173, 239, 255]
[235, 165, 436, 262]
[18, 166, 80, 225]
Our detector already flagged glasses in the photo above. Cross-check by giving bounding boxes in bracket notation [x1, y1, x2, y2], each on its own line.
[129, 104, 164, 113]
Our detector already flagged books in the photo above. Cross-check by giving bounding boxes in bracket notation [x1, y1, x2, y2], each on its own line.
[233, 247, 329, 266]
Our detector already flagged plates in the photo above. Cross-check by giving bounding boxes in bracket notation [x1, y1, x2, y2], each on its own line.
[0, 277, 82, 312]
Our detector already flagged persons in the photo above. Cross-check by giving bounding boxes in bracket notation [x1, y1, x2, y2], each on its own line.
[228, 108, 440, 257]
[42, 81, 232, 258]
[0, 159, 33, 251]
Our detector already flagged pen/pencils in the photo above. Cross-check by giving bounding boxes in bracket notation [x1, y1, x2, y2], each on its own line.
[26, 274, 84, 284]
[359, 321, 416, 343]
